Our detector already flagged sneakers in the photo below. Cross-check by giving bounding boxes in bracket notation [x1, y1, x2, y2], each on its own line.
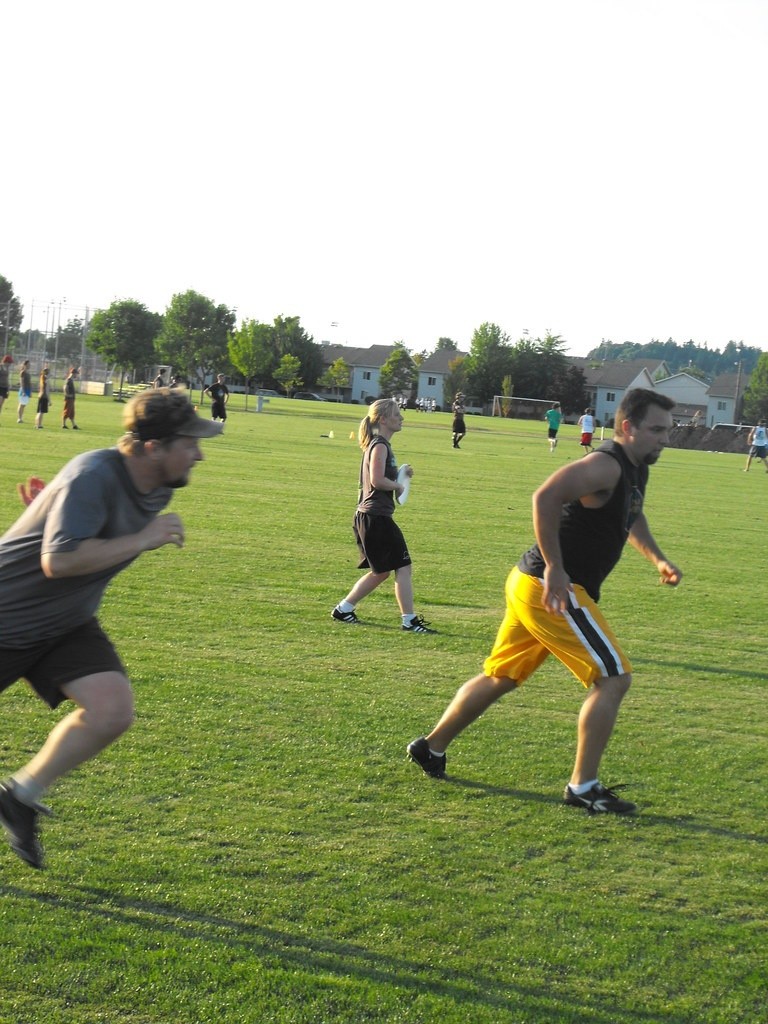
[0, 782, 50, 866]
[563, 784, 637, 814]
[404, 736, 452, 782]
[401, 614, 437, 633]
[331, 605, 361, 623]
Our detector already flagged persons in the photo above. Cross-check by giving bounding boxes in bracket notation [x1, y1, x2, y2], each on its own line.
[17, 360, 31, 422]
[153, 369, 166, 387]
[34, 368, 50, 430]
[330, 399, 437, 634]
[0, 388, 224, 870]
[0, 354, 14, 412]
[744, 419, 768, 473]
[544, 403, 561, 453]
[203, 373, 230, 423]
[62, 368, 79, 429]
[452, 392, 466, 448]
[406, 389, 683, 814]
[392, 395, 436, 413]
[578, 408, 595, 453]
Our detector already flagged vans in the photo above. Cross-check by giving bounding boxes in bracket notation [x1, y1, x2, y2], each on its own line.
[711, 422, 755, 442]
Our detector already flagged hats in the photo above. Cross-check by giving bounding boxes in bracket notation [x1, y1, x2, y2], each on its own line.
[455, 394, 467, 399]
[70, 368, 80, 374]
[124, 388, 224, 442]
[3, 355, 15, 363]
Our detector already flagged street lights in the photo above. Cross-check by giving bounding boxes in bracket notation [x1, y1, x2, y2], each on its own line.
[732, 359, 743, 424]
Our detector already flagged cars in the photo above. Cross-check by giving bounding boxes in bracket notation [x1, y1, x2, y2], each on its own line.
[256, 389, 287, 398]
[293, 391, 328, 402]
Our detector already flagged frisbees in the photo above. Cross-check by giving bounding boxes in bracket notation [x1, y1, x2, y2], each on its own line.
[395, 463, 411, 505]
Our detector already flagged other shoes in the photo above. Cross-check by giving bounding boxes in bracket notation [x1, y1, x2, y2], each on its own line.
[73, 425, 79, 429]
[452, 444, 461, 448]
[553, 440, 557, 448]
[551, 448, 554, 452]
[62, 426, 68, 429]
[17, 419, 22, 423]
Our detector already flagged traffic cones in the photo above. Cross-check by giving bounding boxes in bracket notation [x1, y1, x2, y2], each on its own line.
[349, 432, 356, 439]
[328, 431, 334, 439]
